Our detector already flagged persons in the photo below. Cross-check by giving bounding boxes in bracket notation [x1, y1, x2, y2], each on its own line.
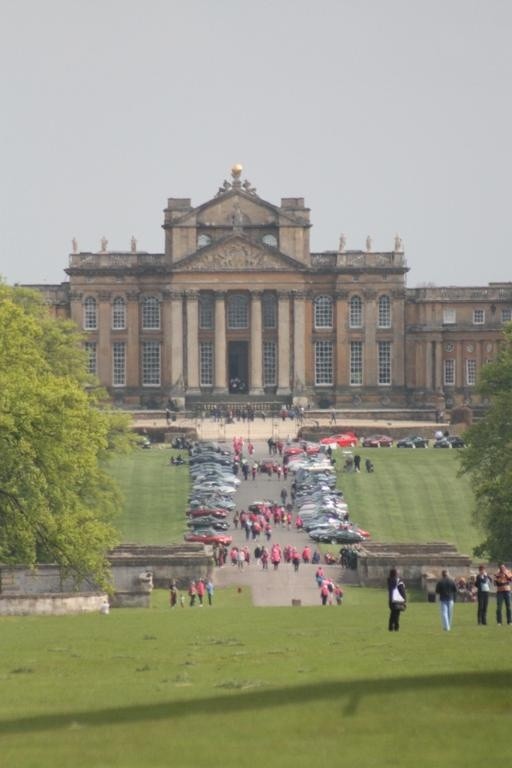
[280, 405, 303, 421]
[296, 515, 303, 530]
[266, 463, 288, 481]
[232, 435, 253, 458]
[214, 542, 251, 572]
[233, 501, 293, 542]
[440, 409, 443, 423]
[189, 578, 214, 606]
[427, 564, 512, 631]
[280, 487, 288, 505]
[211, 401, 255, 424]
[301, 546, 321, 564]
[267, 437, 283, 458]
[326, 544, 360, 571]
[331, 407, 337, 425]
[316, 567, 344, 606]
[170, 584, 177, 606]
[254, 544, 301, 572]
[436, 409, 441, 423]
[326, 446, 372, 474]
[232, 455, 257, 481]
[388, 569, 406, 632]
[169, 434, 193, 467]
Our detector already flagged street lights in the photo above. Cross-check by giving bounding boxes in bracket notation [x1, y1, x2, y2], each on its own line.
[246, 401, 251, 438]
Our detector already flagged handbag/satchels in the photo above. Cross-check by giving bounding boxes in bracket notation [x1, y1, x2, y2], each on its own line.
[392, 587, 405, 603]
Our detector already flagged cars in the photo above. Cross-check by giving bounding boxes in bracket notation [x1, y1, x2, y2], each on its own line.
[128, 436, 150, 448]
[397, 434, 429, 448]
[321, 432, 357, 447]
[434, 435, 465, 448]
[171, 436, 197, 448]
[185, 443, 241, 543]
[248, 501, 284, 514]
[363, 433, 393, 448]
[283, 439, 371, 543]
[260, 457, 279, 471]
